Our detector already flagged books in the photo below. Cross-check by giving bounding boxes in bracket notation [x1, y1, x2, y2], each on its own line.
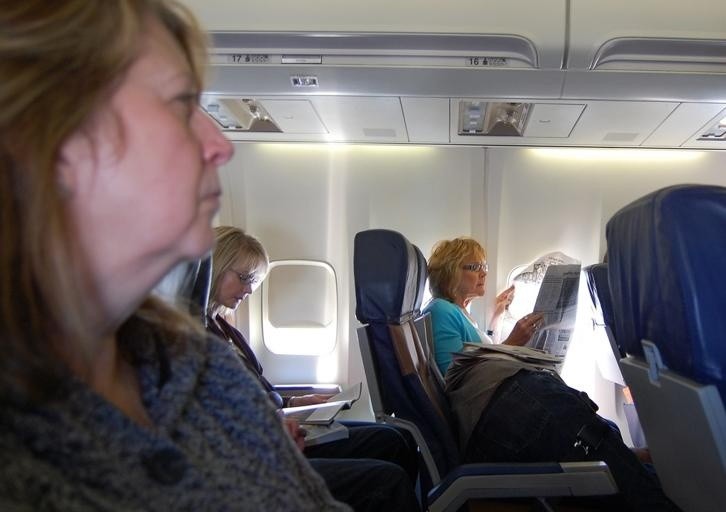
[290, 417, 362, 446]
[275, 381, 363, 426]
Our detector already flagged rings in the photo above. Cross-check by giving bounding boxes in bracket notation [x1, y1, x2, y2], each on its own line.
[534, 331, 537, 334]
[534, 327, 538, 331]
[523, 316, 529, 319]
[532, 323, 536, 327]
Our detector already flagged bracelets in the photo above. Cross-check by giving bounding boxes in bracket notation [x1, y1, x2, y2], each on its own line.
[486, 329, 497, 337]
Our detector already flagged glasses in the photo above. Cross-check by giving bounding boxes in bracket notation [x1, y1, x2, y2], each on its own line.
[229, 269, 257, 285]
[457, 263, 489, 272]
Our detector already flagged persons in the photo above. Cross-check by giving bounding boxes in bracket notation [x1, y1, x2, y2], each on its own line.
[422, 235, 657, 476]
[0, 0, 351, 512]
[206, 225, 419, 493]
[279, 416, 423, 512]
[444, 343, 669, 512]
